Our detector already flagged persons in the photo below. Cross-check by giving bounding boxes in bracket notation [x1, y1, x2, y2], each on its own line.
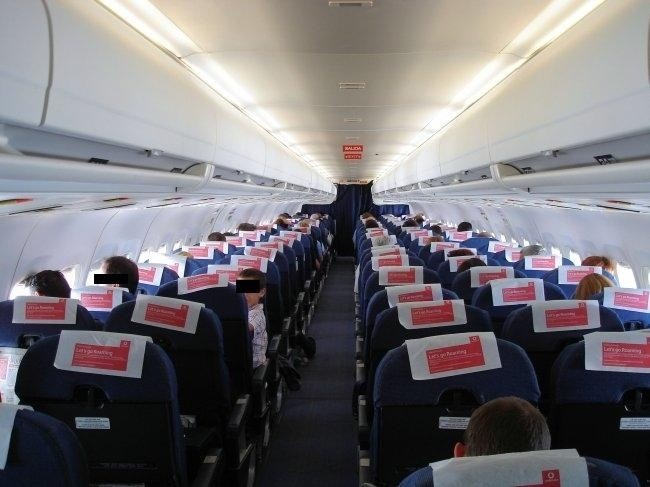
[8, 269, 72, 299]
[98, 254, 139, 296]
[206, 210, 329, 270]
[361, 209, 615, 302]
[236, 269, 268, 377]
[452, 397, 552, 460]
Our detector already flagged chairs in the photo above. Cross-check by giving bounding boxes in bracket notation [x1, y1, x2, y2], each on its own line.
[352, 213, 649, 486]
[0, 210, 339, 486]
